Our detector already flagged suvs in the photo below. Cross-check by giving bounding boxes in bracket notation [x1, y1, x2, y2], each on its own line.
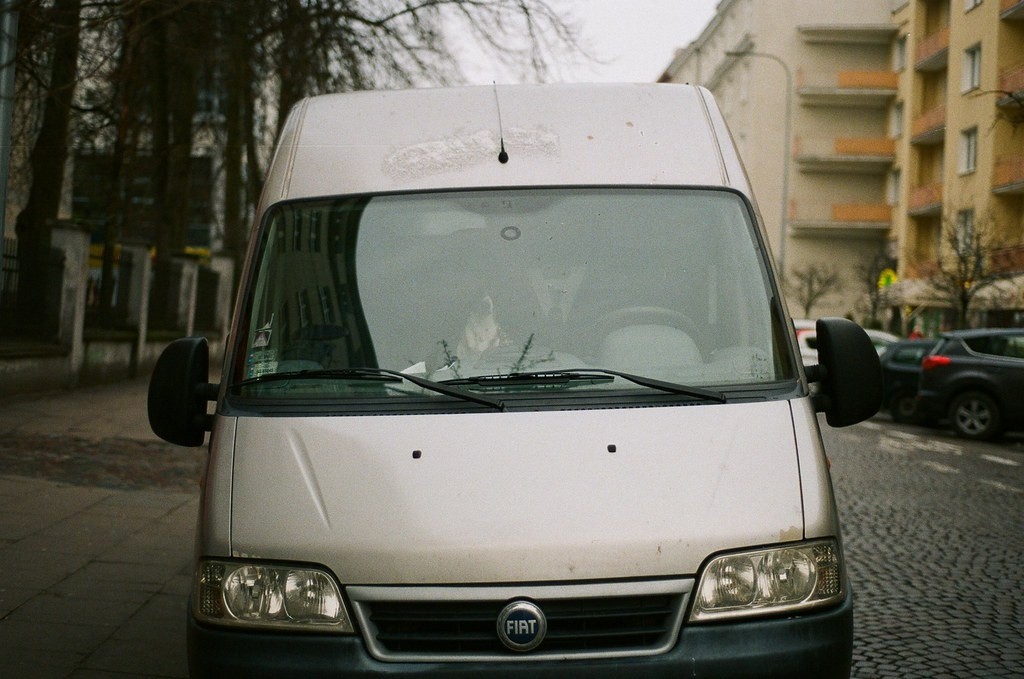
[919, 327, 1024, 442]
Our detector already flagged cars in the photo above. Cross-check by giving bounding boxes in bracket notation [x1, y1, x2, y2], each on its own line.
[878, 339, 934, 423]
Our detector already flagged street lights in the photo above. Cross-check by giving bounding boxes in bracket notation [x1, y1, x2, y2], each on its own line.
[727, 49, 794, 289]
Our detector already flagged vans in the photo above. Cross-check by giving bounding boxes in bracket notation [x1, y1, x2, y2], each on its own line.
[144, 82, 885, 679]
